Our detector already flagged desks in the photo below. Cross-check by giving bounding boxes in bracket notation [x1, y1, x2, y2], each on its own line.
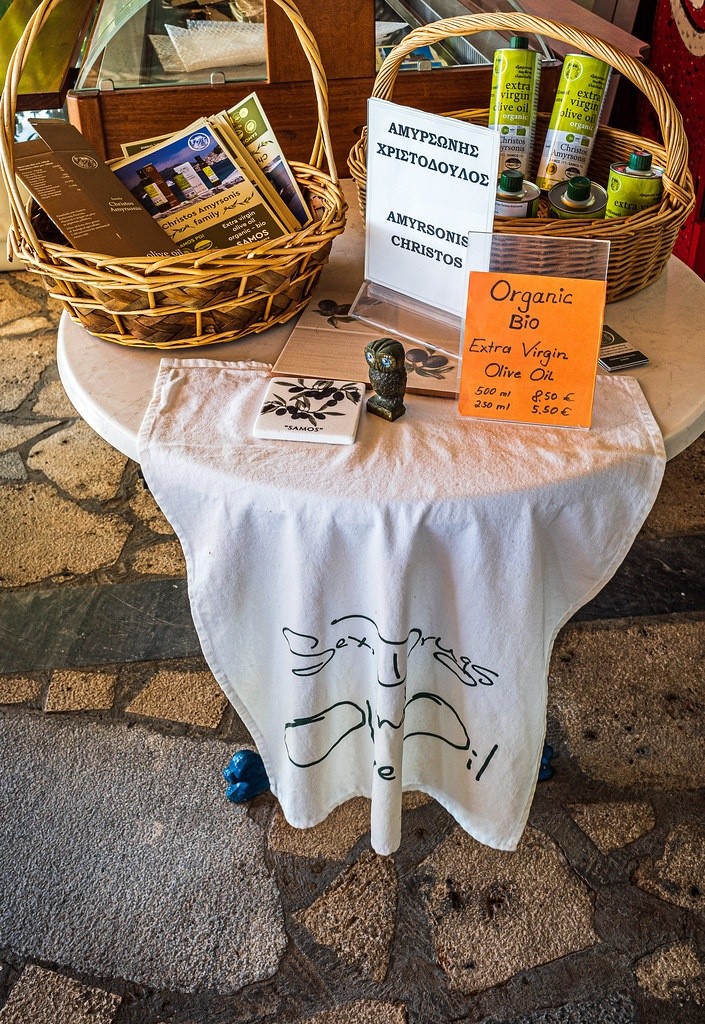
[57, 177, 705, 857]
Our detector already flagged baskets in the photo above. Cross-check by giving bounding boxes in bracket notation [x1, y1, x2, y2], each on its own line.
[2, 0, 350, 350]
[346, 11, 696, 304]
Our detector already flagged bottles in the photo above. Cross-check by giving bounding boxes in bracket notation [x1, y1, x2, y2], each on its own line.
[487, 35, 665, 222]
